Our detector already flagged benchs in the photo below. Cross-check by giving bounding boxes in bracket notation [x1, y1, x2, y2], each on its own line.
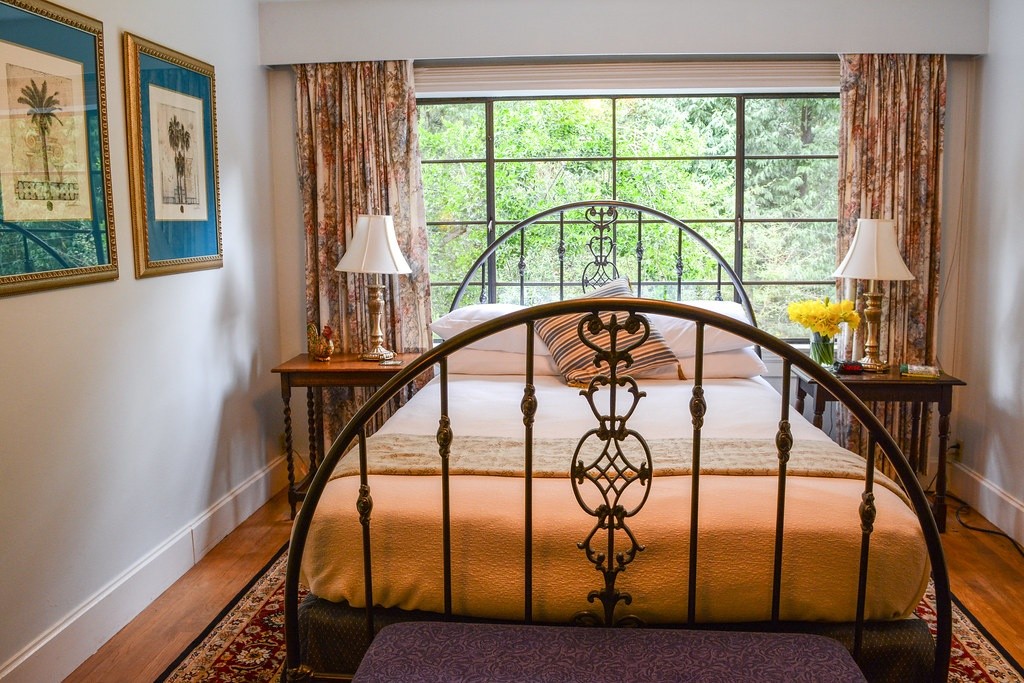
[351, 619, 867, 683]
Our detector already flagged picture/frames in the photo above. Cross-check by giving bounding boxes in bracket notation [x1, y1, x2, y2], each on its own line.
[122, 30, 223, 280]
[0, 0, 119, 299]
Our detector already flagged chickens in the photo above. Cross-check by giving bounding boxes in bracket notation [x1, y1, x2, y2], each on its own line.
[307, 323, 336, 362]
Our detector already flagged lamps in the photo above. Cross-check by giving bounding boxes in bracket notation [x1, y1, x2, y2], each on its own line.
[335, 214, 413, 362]
[831, 218, 915, 373]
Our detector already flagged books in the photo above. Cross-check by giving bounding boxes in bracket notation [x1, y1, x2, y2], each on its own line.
[900, 364, 940, 379]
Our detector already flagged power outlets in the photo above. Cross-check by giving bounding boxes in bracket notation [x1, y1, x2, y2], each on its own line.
[952, 436, 965, 461]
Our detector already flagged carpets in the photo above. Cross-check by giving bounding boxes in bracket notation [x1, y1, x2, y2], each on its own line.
[154, 540, 1024, 683]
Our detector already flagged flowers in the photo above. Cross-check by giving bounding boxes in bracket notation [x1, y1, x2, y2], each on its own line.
[786, 294, 861, 367]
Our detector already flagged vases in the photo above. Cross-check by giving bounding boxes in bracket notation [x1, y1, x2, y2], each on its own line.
[810, 326, 835, 372]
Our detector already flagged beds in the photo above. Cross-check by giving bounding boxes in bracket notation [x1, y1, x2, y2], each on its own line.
[279, 201, 952, 683]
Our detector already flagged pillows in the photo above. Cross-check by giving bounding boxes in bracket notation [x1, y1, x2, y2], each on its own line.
[533, 273, 687, 390]
[447, 340, 559, 376]
[677, 347, 768, 378]
[427, 304, 554, 356]
[641, 300, 754, 355]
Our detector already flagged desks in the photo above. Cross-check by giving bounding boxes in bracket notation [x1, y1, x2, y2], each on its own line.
[790, 364, 967, 533]
[271, 353, 423, 521]
